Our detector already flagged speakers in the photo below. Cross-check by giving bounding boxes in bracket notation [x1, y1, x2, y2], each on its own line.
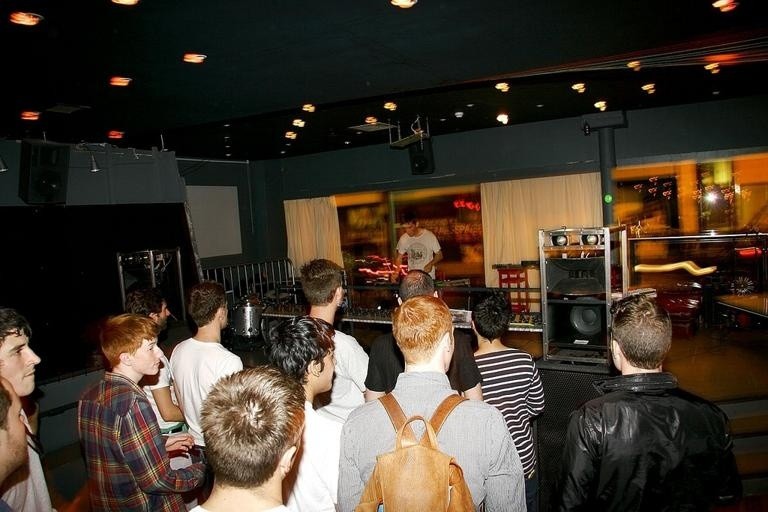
[408, 139, 435, 176]
[18, 136, 72, 204]
[533, 361, 631, 511]
[547, 298, 606, 351]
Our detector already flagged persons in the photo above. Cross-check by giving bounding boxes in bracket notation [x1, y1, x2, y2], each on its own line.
[0, 308, 58, 512]
[0, 375, 28, 512]
[390, 216, 443, 289]
[555, 296, 738, 512]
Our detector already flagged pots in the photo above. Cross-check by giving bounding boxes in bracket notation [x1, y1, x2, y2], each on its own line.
[231, 302, 266, 340]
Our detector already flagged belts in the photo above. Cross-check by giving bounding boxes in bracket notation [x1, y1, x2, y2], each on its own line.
[161, 426, 182, 434]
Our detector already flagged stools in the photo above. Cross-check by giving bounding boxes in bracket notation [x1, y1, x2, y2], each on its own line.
[498, 268, 530, 313]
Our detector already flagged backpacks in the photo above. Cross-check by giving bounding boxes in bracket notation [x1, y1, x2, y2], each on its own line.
[354, 393, 474, 512]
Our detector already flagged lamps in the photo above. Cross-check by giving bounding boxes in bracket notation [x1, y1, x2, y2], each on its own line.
[79, 144, 101, 173]
